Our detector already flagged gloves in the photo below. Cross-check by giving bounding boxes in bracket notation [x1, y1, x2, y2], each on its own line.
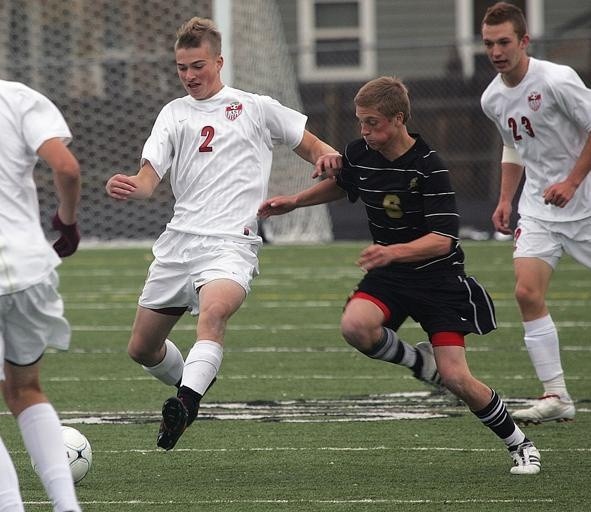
[50, 208, 81, 260]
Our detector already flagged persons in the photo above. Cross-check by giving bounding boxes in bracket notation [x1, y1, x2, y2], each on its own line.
[254, 74, 543, 476]
[0, 78, 87, 512]
[477, 1, 591, 426]
[105, 16, 346, 452]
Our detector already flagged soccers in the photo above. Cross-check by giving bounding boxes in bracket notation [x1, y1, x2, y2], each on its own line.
[30, 425, 93, 484]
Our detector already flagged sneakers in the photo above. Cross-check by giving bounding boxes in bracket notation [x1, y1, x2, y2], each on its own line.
[505, 439, 542, 476]
[414, 340, 461, 403]
[508, 391, 577, 427]
[153, 395, 189, 453]
